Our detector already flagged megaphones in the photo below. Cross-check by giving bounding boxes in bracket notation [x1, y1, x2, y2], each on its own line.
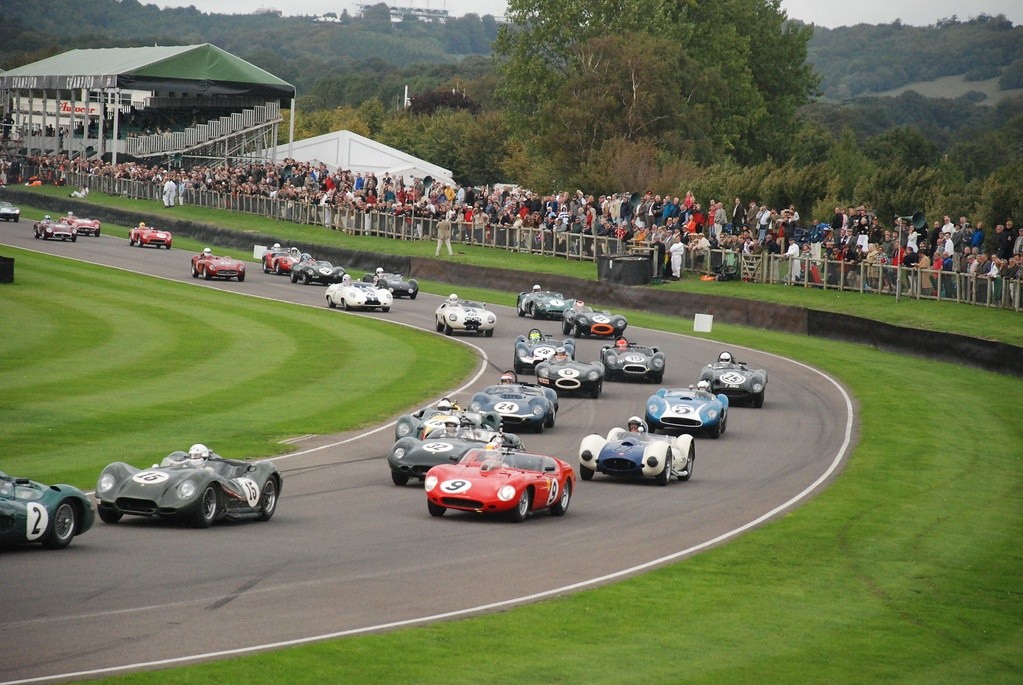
[417, 175, 432, 188]
[622, 191, 641, 206]
[277, 165, 292, 175]
[86, 146, 94, 155]
[900, 211, 926, 228]
[170, 152, 183, 163]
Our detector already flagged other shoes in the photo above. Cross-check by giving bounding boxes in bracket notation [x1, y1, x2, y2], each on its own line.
[661, 267, 1023, 313]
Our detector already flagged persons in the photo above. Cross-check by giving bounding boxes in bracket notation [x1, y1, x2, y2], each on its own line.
[650, 236, 665, 282]
[615, 336, 628, 351]
[169, 178, 177, 206]
[2, 102, 226, 139]
[628, 416, 644, 432]
[555, 348, 568, 364]
[528, 330, 541, 346]
[442, 416, 466, 438]
[498, 374, 514, 392]
[68, 185, 89, 198]
[719, 353, 731, 366]
[666, 236, 684, 281]
[162, 178, 170, 208]
[437, 401, 453, 415]
[486, 442, 510, 468]
[0, 153, 1022, 311]
[697, 380, 709, 391]
[189, 444, 215, 473]
[435, 215, 453, 256]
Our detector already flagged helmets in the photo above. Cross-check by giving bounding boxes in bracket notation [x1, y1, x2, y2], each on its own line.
[485, 442, 501, 460]
[376, 267, 383, 273]
[555, 347, 566, 356]
[290, 247, 298, 255]
[533, 285, 541, 292]
[628, 416, 643, 431]
[697, 381, 710, 391]
[529, 330, 540, 341]
[438, 400, 452, 410]
[204, 248, 212, 256]
[501, 373, 514, 385]
[301, 253, 311, 261]
[188, 444, 209, 460]
[449, 293, 459, 304]
[139, 222, 145, 228]
[615, 338, 627, 346]
[576, 301, 585, 308]
[67, 211, 73, 215]
[274, 243, 280, 248]
[718, 352, 731, 366]
[44, 215, 51, 220]
[443, 416, 460, 432]
[342, 274, 351, 282]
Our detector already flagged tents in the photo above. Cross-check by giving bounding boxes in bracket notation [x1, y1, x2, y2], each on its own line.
[0, 43, 296, 165]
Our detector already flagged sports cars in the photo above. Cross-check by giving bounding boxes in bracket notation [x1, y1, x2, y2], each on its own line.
[361, 272, 420, 300]
[579, 419, 696, 486]
[517, 292, 578, 320]
[291, 257, 345, 285]
[534, 351, 606, 399]
[561, 301, 628, 339]
[190, 252, 245, 282]
[469, 369, 559, 434]
[512, 328, 576, 374]
[0, 470, 95, 552]
[600, 335, 666, 384]
[325, 279, 393, 313]
[128, 227, 173, 249]
[59, 215, 100, 238]
[0, 201, 21, 223]
[643, 377, 729, 440]
[262, 248, 312, 277]
[424, 434, 576, 524]
[387, 397, 525, 487]
[33, 219, 77, 243]
[435, 299, 497, 337]
[95, 449, 283, 529]
[700, 350, 769, 408]
